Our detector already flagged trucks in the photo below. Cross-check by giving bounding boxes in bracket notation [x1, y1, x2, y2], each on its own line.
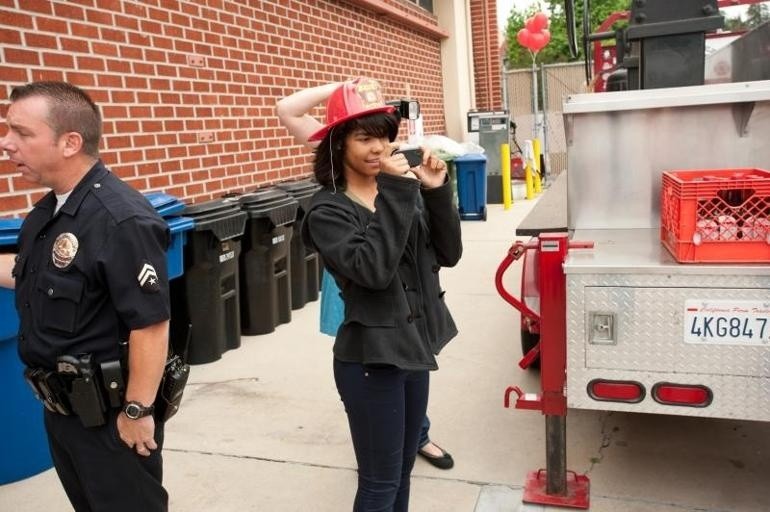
[495, 1, 770, 510]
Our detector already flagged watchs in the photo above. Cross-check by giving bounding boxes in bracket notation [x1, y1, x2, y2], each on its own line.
[120, 401, 157, 421]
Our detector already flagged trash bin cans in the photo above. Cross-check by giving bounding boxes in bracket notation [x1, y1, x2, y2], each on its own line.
[138, 193, 193, 281]
[1, 219, 55, 487]
[170, 177, 325, 365]
[431, 150, 486, 221]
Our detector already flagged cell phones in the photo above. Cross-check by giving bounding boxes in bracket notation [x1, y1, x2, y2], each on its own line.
[395, 148, 424, 168]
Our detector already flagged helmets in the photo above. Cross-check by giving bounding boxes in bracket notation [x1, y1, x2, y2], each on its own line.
[306, 76, 396, 142]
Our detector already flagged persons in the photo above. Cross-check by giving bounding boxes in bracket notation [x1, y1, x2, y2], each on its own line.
[299, 80, 463, 512]
[0, 80, 171, 511]
[274, 76, 454, 468]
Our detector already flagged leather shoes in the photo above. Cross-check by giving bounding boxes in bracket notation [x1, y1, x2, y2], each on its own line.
[418, 440, 453, 469]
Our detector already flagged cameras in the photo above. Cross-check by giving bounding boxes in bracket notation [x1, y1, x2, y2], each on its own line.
[385, 100, 420, 120]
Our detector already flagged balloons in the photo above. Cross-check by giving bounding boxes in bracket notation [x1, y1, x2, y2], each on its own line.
[516, 12, 551, 53]
